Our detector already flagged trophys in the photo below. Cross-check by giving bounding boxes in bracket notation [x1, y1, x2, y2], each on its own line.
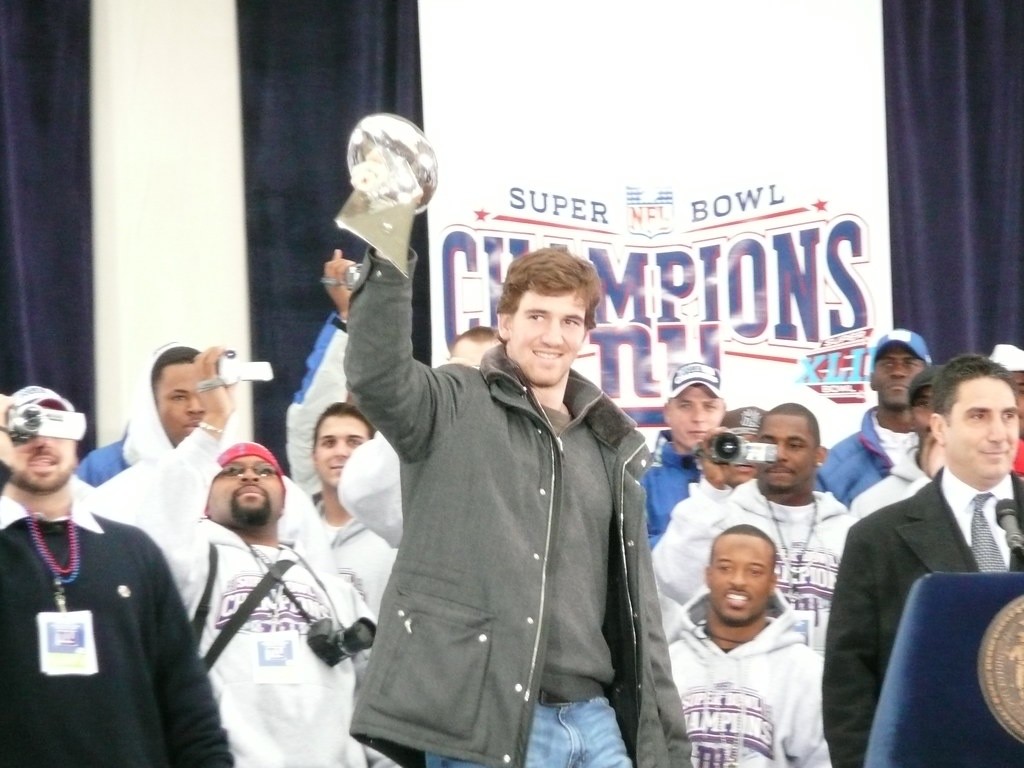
[335, 111, 439, 279]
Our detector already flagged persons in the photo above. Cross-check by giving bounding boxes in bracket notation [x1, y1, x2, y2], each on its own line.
[342, 246, 694, 767]
[70, 328, 1024, 768]
[665, 524, 832, 768]
[0, 384, 242, 768]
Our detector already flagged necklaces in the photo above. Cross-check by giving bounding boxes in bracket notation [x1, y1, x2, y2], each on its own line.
[696, 623, 749, 644]
[246, 535, 284, 619]
[764, 494, 818, 592]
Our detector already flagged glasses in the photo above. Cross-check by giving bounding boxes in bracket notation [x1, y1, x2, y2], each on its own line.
[216, 461, 278, 479]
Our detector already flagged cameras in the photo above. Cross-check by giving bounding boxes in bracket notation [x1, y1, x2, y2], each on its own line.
[306, 617, 379, 668]
[217, 348, 274, 386]
[707, 431, 778, 463]
[6, 403, 87, 442]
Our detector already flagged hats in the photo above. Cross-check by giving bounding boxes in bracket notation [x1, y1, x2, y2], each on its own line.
[10, 384, 75, 413]
[869, 329, 931, 370]
[666, 364, 725, 400]
[989, 344, 1024, 372]
[218, 441, 283, 483]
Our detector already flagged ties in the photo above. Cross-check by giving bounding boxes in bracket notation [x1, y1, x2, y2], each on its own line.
[968, 494, 1008, 574]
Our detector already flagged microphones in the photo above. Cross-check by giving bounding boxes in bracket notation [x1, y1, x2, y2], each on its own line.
[996, 498, 1024, 555]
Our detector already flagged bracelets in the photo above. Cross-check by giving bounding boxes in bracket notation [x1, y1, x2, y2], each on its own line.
[196, 420, 225, 434]
